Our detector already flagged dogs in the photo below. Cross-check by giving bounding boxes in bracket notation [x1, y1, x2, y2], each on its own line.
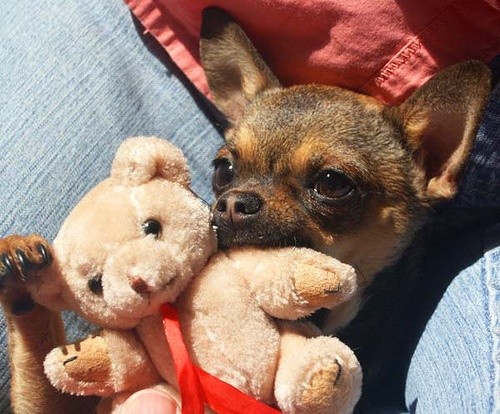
[0, 4, 496, 412]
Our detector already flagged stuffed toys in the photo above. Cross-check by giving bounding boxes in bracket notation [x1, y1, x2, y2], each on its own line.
[26, 134, 365, 413]
